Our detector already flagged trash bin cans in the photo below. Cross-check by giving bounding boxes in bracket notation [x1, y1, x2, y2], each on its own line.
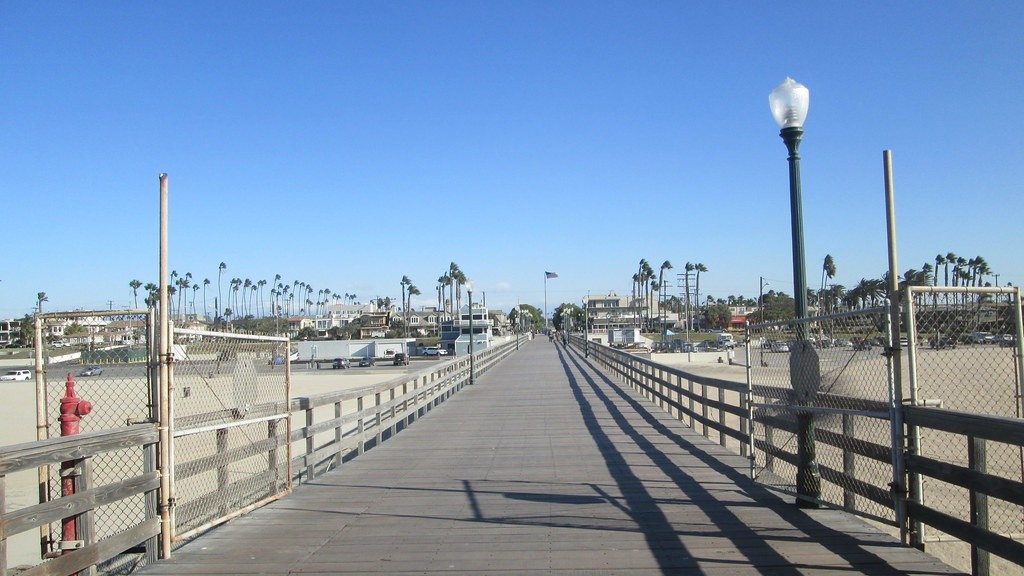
[183, 387, 190, 398]
[528, 335, 531, 341]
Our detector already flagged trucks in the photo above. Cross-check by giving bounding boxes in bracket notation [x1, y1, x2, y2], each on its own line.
[384, 349, 397, 360]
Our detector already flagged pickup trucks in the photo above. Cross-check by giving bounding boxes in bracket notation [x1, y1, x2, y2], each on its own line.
[51, 341, 71, 348]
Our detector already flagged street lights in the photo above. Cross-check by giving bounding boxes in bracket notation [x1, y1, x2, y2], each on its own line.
[463, 276, 475, 384]
[768, 75, 823, 508]
[581, 294, 590, 359]
[515, 305, 520, 351]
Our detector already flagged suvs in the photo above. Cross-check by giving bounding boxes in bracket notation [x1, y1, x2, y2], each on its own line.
[422, 346, 448, 357]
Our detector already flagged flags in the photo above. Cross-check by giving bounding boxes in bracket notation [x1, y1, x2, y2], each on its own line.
[546, 272, 558, 279]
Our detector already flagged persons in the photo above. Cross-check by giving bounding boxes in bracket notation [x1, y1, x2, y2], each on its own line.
[548, 329, 569, 346]
[760, 336, 766, 349]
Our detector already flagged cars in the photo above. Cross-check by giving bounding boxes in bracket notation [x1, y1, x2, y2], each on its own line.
[0, 370, 32, 382]
[393, 353, 408, 367]
[4, 343, 23, 349]
[332, 357, 351, 369]
[900, 337, 909, 346]
[915, 332, 1018, 351]
[80, 365, 103, 378]
[653, 332, 746, 352]
[358, 357, 376, 367]
[272, 356, 286, 366]
[763, 335, 886, 353]
